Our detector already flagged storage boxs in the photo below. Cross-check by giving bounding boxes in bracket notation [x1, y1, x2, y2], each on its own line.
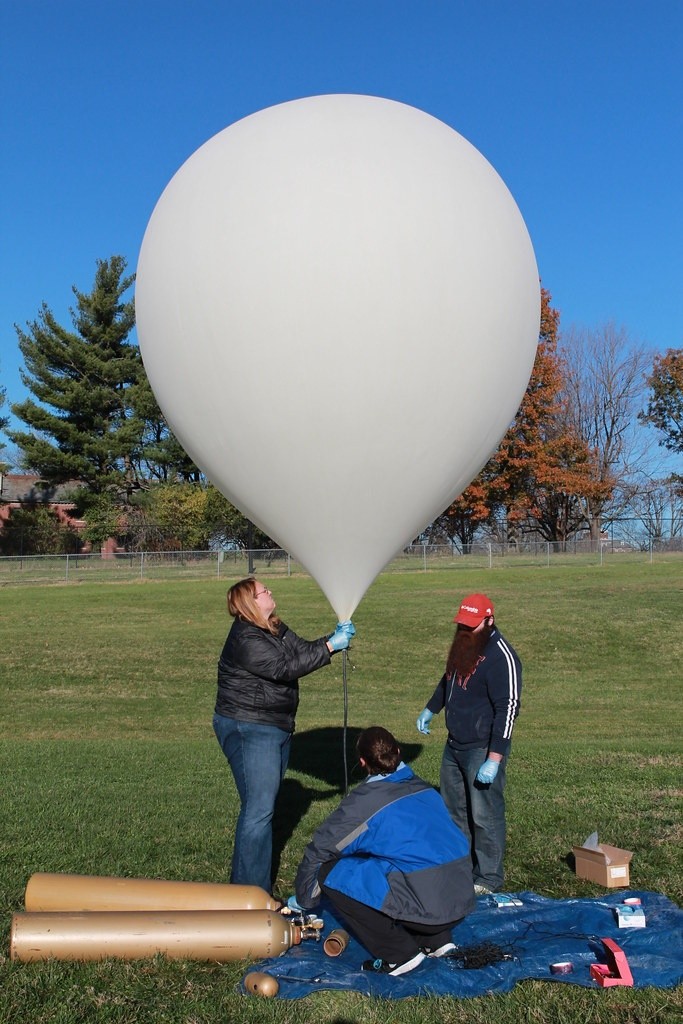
[574, 843, 634, 888]
[591, 939, 635, 987]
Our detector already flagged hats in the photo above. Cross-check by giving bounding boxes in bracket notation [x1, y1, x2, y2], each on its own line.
[452, 592, 493, 627]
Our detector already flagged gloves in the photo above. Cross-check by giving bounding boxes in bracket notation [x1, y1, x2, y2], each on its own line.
[329, 622, 356, 649]
[287, 895, 305, 912]
[477, 758, 500, 784]
[417, 708, 433, 736]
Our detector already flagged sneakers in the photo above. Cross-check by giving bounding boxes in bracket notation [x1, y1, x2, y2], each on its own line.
[474, 872, 512, 893]
[423, 932, 458, 957]
[362, 947, 425, 974]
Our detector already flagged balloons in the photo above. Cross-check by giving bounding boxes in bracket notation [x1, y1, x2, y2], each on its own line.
[135, 93, 541, 626]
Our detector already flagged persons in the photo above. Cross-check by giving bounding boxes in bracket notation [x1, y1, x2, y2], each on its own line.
[287, 726, 473, 978]
[416, 594, 522, 896]
[213, 577, 356, 903]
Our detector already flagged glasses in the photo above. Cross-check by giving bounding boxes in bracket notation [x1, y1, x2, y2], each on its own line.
[254, 588, 268, 596]
